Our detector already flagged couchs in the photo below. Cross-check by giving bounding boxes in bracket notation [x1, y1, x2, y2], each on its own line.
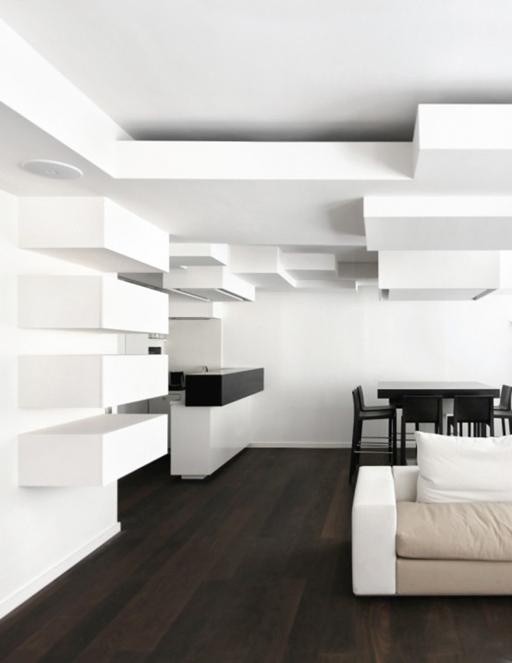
[353, 428, 512, 597]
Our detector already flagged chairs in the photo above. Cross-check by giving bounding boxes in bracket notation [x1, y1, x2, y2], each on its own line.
[348, 383, 512, 483]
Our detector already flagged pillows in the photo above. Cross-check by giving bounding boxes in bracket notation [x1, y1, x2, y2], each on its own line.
[409, 424, 512, 502]
